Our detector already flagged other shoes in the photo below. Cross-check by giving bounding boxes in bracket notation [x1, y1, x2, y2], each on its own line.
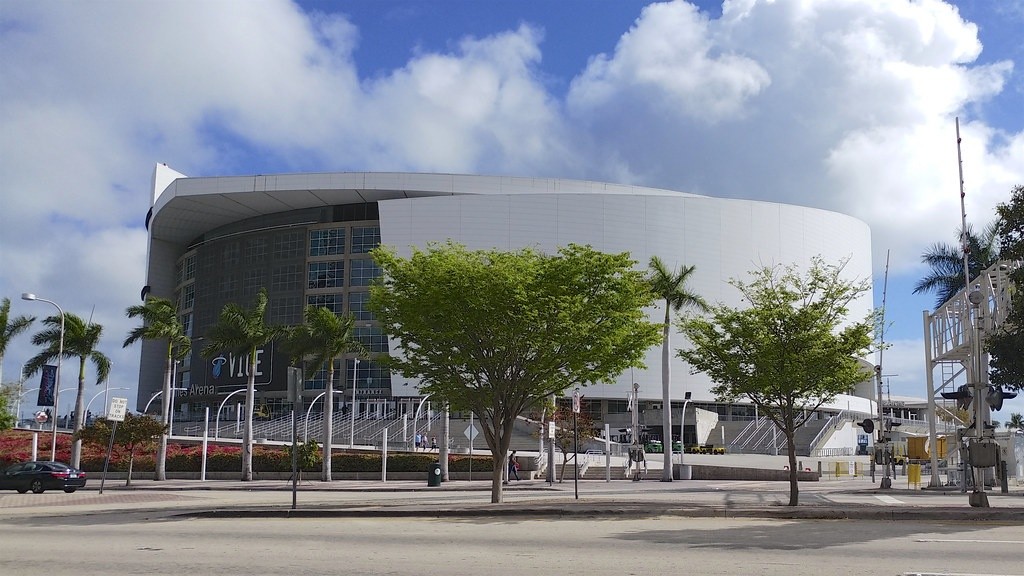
[508, 480, 512, 482]
[517, 478, 522, 481]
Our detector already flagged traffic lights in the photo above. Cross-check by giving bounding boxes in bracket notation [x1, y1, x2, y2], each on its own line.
[939, 386, 972, 409]
[857, 420, 874, 434]
[986, 385, 1018, 411]
[886, 419, 901, 433]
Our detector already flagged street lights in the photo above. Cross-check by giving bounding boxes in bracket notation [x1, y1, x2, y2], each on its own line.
[83, 388, 131, 428]
[304, 391, 344, 445]
[143, 388, 188, 415]
[215, 388, 258, 441]
[21, 293, 64, 462]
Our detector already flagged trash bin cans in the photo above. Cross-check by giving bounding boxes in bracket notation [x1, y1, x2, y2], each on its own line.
[427, 462, 442, 486]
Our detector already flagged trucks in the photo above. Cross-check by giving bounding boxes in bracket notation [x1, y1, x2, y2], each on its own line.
[700, 444, 726, 454]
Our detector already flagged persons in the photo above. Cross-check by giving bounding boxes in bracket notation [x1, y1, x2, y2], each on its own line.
[415, 430, 422, 451]
[509, 449, 520, 481]
[420, 432, 428, 451]
[429, 433, 437, 452]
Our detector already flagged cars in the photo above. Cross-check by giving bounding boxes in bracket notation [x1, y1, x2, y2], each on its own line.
[0, 461, 88, 494]
[645, 443, 663, 453]
